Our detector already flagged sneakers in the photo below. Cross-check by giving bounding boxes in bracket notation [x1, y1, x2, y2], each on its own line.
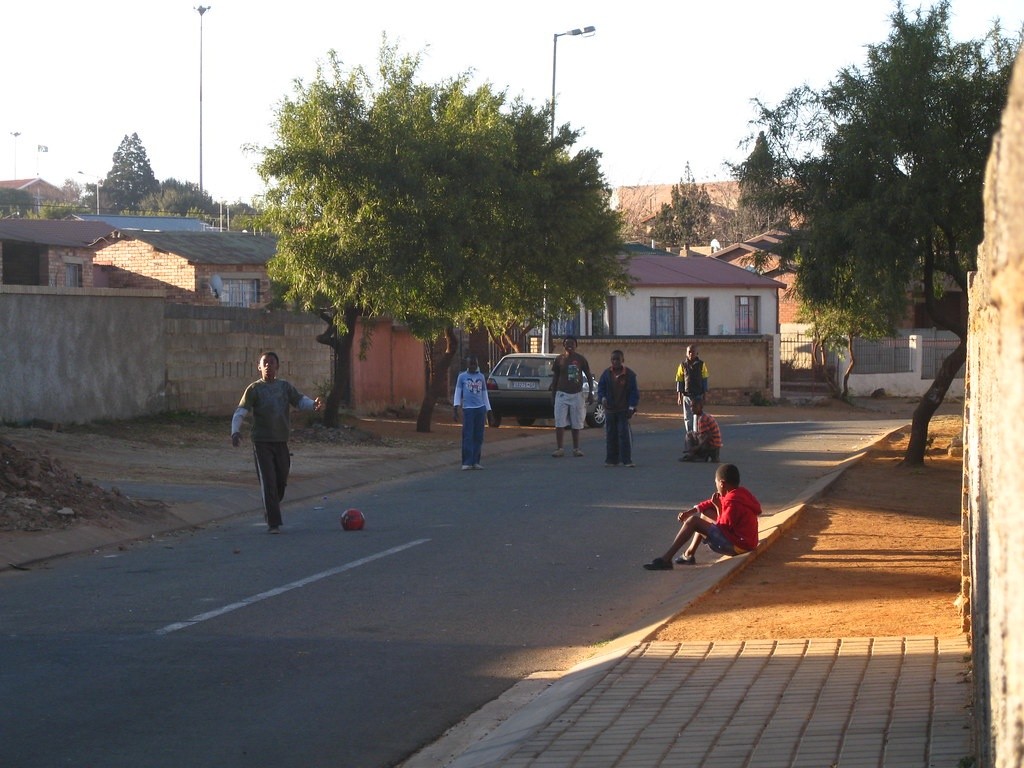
[643, 557, 673, 570]
[675, 553, 695, 565]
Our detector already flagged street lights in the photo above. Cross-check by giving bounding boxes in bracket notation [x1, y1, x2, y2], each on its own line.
[196, 3, 212, 222]
[540, 26, 598, 353]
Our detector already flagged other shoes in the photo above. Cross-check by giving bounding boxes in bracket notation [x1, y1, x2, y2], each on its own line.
[679, 455, 696, 462]
[605, 463, 617, 467]
[573, 448, 583, 456]
[472, 464, 485, 470]
[625, 463, 636, 467]
[551, 448, 565, 456]
[269, 526, 281, 533]
[710, 447, 720, 462]
[461, 465, 472, 470]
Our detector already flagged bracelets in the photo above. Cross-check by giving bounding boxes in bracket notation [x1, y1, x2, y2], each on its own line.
[629, 406, 634, 410]
[590, 391, 594, 395]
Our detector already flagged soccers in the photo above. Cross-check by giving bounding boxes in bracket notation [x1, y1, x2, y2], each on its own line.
[340, 509, 365, 530]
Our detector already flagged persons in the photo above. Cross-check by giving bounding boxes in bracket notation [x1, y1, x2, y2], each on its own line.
[598, 349, 640, 467]
[552, 336, 595, 457]
[231, 351, 323, 534]
[679, 398, 724, 463]
[676, 344, 710, 453]
[453, 354, 494, 470]
[643, 463, 762, 570]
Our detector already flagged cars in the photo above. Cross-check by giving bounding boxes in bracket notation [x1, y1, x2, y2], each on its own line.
[487, 353, 606, 427]
